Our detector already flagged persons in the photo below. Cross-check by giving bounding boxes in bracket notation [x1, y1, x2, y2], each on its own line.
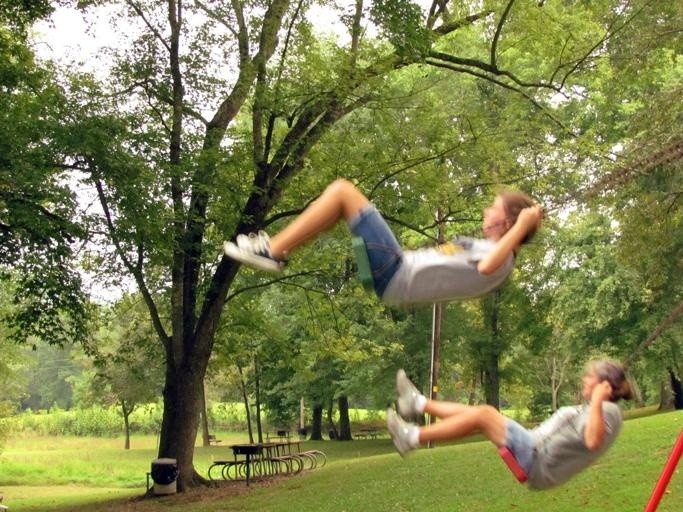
[385, 357, 638, 491]
[223, 178, 546, 308]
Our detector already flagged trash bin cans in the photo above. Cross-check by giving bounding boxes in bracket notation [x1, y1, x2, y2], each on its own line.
[151, 459, 177, 494]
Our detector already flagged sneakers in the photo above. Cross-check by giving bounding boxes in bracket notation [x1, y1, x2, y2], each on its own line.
[386, 407, 416, 457]
[396, 369, 419, 415]
[223, 232, 289, 276]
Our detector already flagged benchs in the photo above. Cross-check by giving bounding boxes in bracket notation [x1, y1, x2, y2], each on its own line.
[204, 434, 222, 446]
[208, 448, 327, 480]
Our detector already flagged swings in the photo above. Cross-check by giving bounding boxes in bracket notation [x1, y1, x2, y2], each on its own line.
[497, 299, 682, 484]
[351, 139, 683, 292]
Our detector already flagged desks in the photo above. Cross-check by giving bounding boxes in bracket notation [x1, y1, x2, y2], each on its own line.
[230, 438, 303, 486]
